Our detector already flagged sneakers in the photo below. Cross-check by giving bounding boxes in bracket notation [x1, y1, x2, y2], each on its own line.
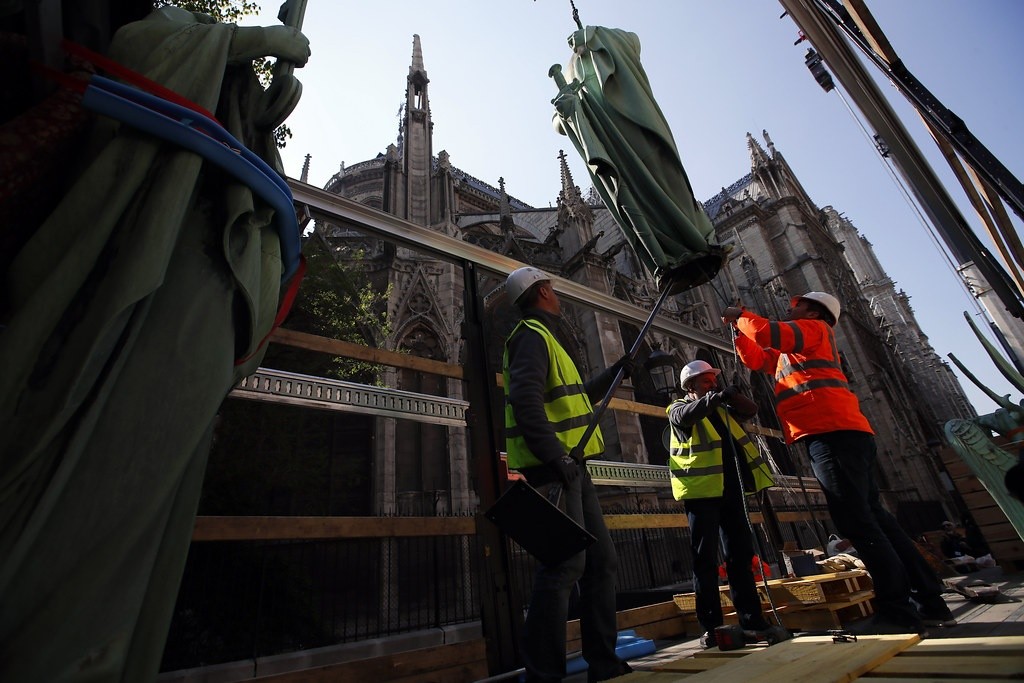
[917, 598, 957, 627]
[850, 604, 929, 639]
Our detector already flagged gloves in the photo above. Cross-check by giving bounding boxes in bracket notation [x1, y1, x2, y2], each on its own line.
[550, 450, 582, 494]
[612, 353, 636, 379]
[705, 385, 737, 402]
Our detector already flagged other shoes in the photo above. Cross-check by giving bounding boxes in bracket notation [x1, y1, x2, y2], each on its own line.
[743, 626, 781, 641]
[587, 658, 633, 682]
[700, 631, 716, 649]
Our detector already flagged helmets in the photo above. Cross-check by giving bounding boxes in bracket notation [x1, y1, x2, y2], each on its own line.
[506, 267, 551, 306]
[790, 292, 840, 326]
[680, 360, 721, 391]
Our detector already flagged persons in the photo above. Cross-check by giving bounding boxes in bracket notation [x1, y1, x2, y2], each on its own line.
[963, 517, 989, 560]
[719, 291, 955, 636]
[0, 0, 315, 682]
[501, 267, 634, 683]
[940, 519, 970, 560]
[666, 361, 785, 649]
[547, 1, 725, 293]
